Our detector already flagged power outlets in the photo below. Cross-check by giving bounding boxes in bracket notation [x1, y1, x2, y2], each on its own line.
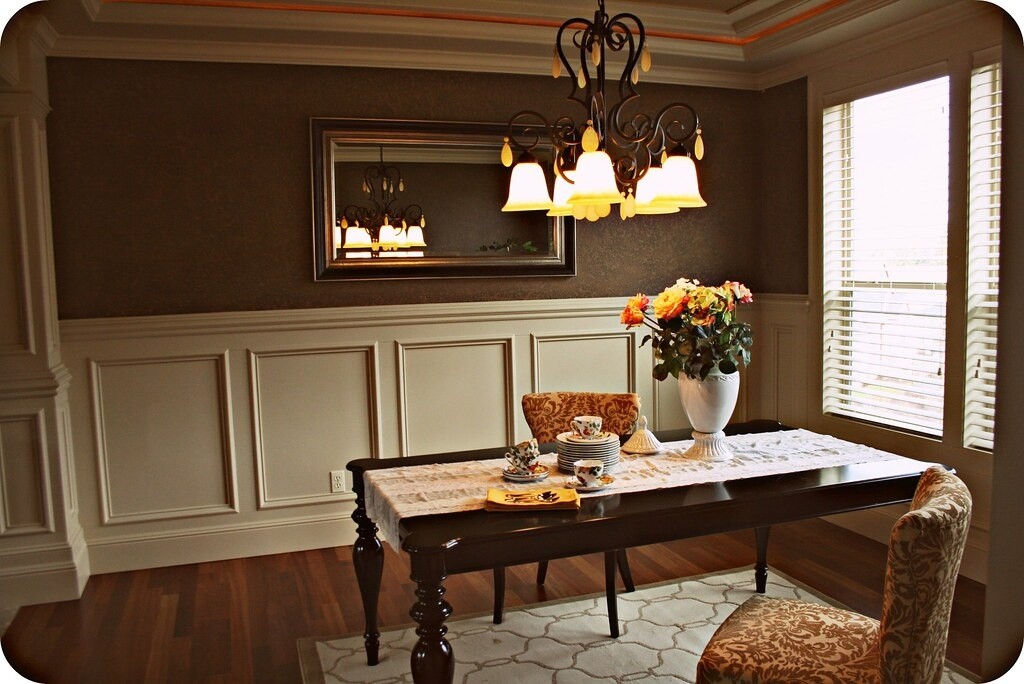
[330, 471, 345, 493]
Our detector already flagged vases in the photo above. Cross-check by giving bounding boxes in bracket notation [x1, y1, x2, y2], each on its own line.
[677, 365, 739, 458]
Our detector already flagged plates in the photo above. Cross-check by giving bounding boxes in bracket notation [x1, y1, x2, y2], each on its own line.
[556, 430, 621, 471]
[564, 474, 617, 491]
[501, 464, 550, 481]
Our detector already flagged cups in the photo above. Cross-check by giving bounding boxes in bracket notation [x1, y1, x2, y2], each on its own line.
[570, 415, 602, 439]
[574, 459, 604, 486]
[505, 438, 540, 474]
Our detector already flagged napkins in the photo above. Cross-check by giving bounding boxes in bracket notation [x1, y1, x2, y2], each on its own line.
[487, 486, 582, 511]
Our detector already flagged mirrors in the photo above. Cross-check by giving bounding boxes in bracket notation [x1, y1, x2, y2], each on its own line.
[307, 116, 576, 277]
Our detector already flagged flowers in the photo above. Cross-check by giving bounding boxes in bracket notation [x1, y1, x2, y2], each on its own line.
[620, 275, 753, 381]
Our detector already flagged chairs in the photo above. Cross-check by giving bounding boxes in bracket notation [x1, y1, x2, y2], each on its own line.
[492, 392, 639, 640]
[695, 466, 973, 683]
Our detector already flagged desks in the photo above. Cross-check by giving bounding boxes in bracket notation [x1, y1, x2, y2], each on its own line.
[345, 420, 950, 682]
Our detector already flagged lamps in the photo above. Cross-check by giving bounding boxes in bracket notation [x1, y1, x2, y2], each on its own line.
[336, 142, 427, 249]
[498, 0, 708, 223]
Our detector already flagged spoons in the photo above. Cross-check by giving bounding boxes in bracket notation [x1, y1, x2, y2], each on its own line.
[506, 491, 560, 502]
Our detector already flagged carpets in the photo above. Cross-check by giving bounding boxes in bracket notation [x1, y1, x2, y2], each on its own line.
[297, 561, 987, 684]
